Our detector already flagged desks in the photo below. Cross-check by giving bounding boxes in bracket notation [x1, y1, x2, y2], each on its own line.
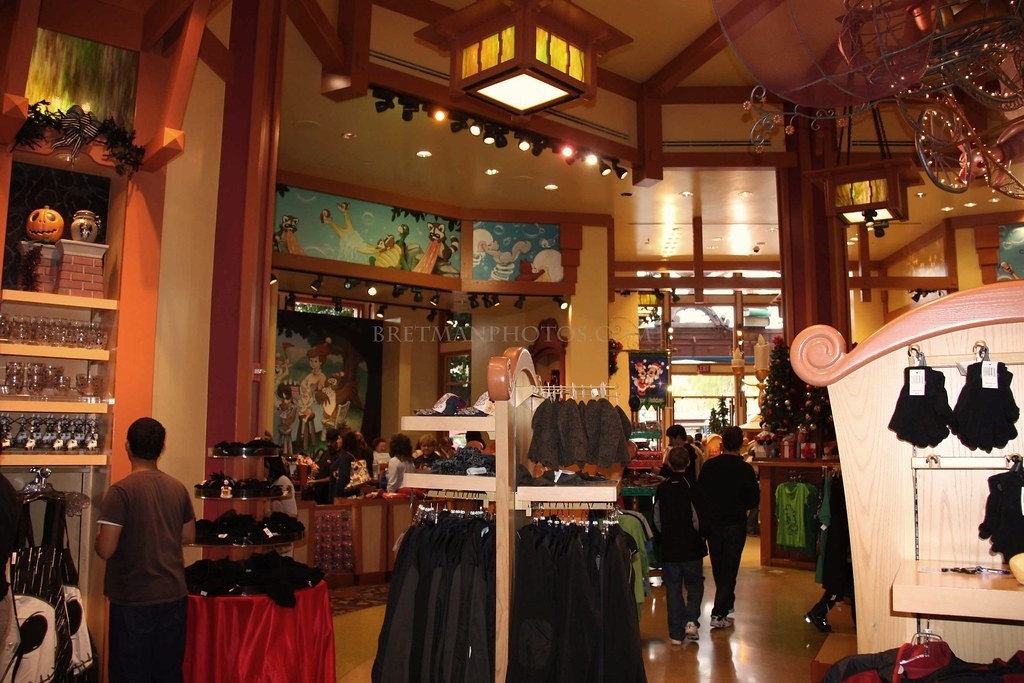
[182, 577, 336, 683]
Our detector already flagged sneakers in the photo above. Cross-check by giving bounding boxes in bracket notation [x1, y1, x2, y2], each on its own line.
[709, 615, 735, 628]
[685, 622, 699, 640]
[671, 638, 682, 644]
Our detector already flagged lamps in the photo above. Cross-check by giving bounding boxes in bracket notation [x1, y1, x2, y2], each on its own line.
[801, 157, 925, 238]
[413, 0, 635, 124]
[375, 99, 628, 181]
[743, 0, 1024, 200]
[285, 274, 568, 325]
[912, 289, 924, 303]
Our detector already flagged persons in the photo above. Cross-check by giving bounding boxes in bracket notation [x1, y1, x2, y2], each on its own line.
[92, 416, 198, 683]
[651, 446, 710, 646]
[800, 463, 856, 633]
[691, 425, 762, 630]
[657, 423, 705, 483]
[303, 420, 497, 503]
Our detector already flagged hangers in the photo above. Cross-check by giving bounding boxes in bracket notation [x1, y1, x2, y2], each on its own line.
[908, 342, 929, 367]
[15, 467, 93, 518]
[899, 613, 952, 668]
[531, 501, 623, 539]
[407, 490, 495, 531]
[996, 452, 1024, 492]
[955, 340, 991, 376]
[787, 465, 841, 484]
[546, 381, 621, 407]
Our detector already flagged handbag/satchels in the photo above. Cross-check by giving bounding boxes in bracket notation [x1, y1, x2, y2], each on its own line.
[1, 496, 97, 683]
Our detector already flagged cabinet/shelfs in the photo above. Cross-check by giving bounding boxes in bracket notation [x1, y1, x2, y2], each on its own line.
[0, 287, 120, 466]
[400, 346, 625, 682]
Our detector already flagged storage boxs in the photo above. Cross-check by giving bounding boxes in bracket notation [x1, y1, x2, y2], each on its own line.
[754, 437, 839, 460]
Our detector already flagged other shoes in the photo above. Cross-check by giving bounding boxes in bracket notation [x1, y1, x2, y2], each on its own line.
[804, 602, 832, 632]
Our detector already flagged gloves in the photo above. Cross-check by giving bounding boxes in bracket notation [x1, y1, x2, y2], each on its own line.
[978, 474, 1024, 561]
[890, 363, 1020, 452]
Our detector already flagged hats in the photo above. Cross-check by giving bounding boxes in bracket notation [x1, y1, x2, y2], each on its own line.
[412, 392, 467, 416]
[454, 391, 496, 417]
